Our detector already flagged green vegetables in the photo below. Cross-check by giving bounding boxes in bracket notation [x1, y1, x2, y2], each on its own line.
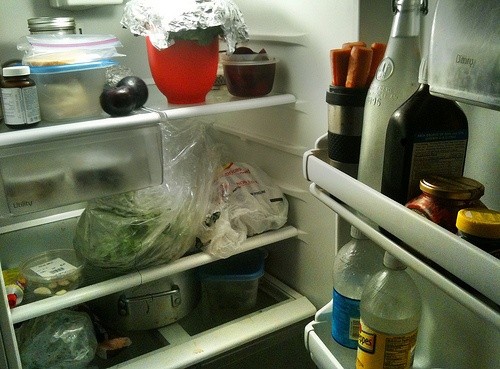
[71, 192, 194, 267]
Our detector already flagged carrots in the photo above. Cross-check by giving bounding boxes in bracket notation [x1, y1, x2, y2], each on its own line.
[329, 40, 385, 88]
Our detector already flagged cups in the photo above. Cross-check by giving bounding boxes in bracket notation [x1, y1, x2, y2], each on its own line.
[145, 33, 219, 105]
[326, 86, 368, 178]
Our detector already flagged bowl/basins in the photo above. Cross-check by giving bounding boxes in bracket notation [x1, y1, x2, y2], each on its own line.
[94, 266, 197, 330]
[220, 54, 276, 97]
[197, 244, 271, 309]
[29, 58, 121, 124]
[20, 246, 90, 295]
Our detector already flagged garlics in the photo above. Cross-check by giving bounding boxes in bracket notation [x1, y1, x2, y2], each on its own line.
[33, 272, 78, 295]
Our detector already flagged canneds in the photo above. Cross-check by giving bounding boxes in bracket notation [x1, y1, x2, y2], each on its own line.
[404, 174, 500, 262]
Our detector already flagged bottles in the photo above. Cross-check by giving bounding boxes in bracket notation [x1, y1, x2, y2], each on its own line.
[357, 0, 429, 228]
[406, 174, 489, 238]
[332, 214, 386, 369]
[1, 66, 42, 129]
[442, 207, 500, 314]
[355, 251, 420, 368]
[28, 15, 79, 35]
[379, 54, 468, 248]
